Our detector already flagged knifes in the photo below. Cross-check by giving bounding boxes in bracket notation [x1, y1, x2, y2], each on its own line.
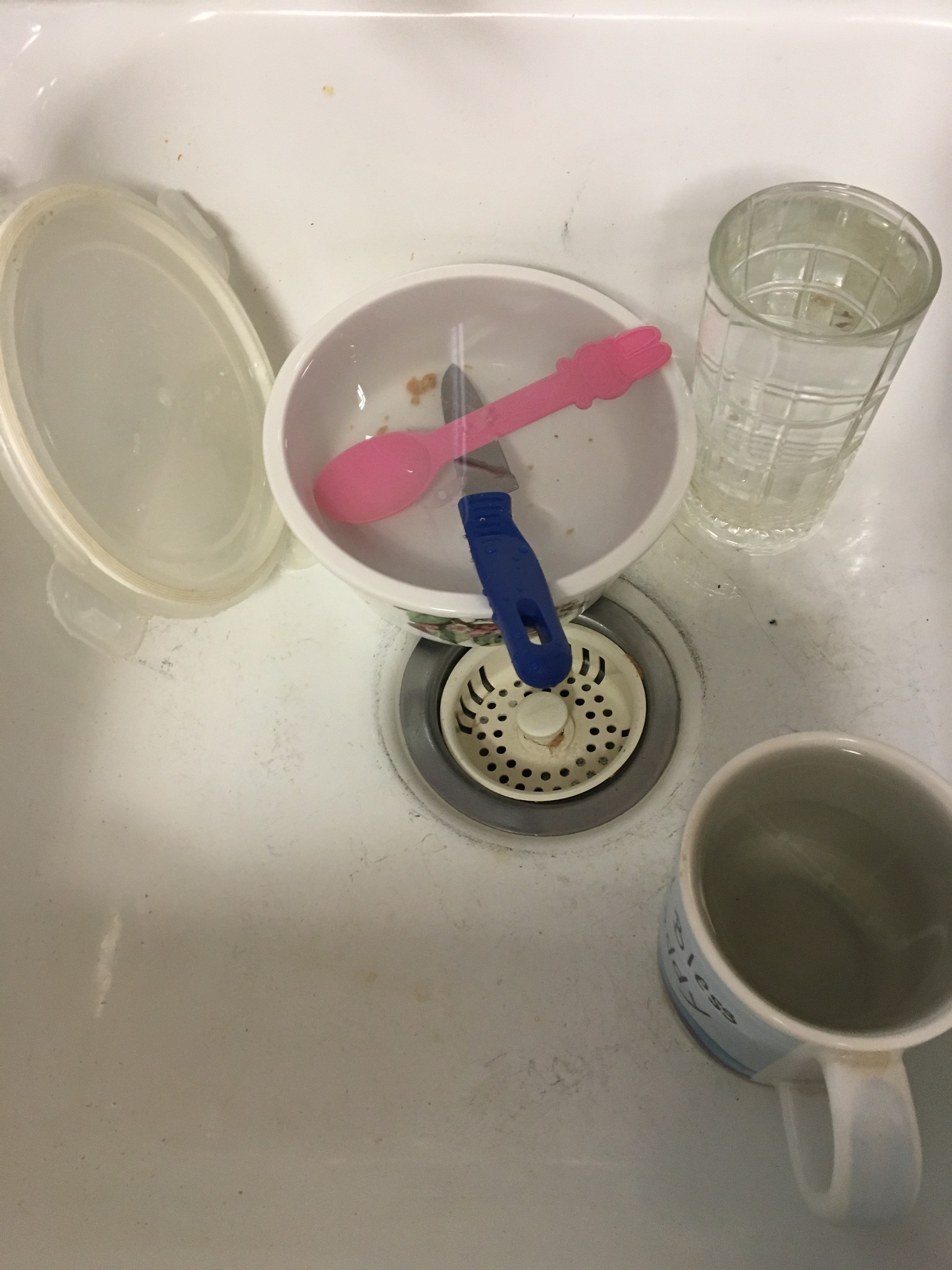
[440, 361, 572, 689]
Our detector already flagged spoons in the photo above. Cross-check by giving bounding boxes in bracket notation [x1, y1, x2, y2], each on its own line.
[312, 325, 672, 525]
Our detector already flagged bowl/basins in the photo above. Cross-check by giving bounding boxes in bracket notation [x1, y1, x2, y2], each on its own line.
[262, 263, 698, 646]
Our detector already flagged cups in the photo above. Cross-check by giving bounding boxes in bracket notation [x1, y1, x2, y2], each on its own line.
[682, 181, 942, 556]
[658, 730, 952, 1222]
